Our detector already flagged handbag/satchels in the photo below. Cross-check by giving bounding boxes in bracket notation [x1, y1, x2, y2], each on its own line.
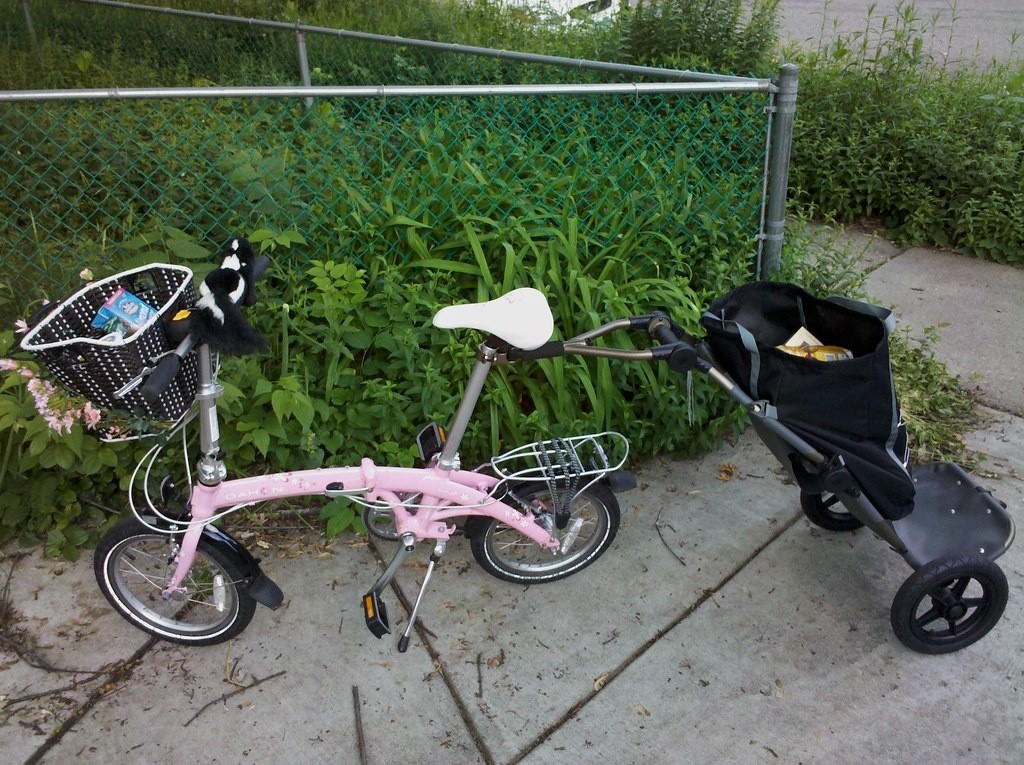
[701, 281, 916, 521]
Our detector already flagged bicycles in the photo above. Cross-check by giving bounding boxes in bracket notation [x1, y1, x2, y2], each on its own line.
[20, 233, 632, 647]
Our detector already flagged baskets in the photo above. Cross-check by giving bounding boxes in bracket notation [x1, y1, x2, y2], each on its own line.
[20, 262, 221, 443]
